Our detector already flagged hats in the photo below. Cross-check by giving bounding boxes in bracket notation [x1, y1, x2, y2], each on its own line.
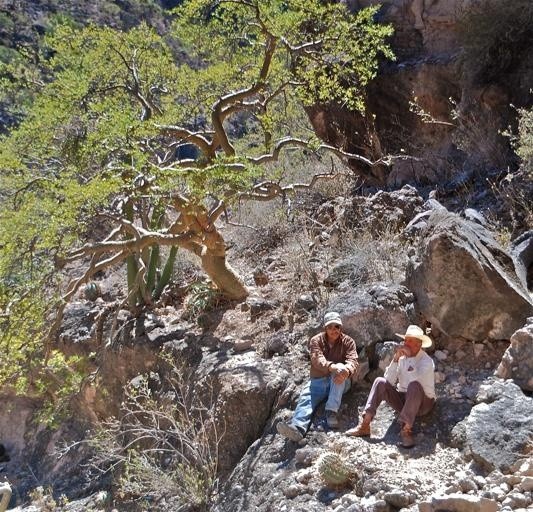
[324, 311, 343, 327]
[395, 324, 433, 348]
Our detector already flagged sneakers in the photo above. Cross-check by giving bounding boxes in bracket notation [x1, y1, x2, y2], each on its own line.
[325, 409, 338, 428]
[345, 423, 371, 436]
[276, 421, 304, 442]
[400, 428, 415, 449]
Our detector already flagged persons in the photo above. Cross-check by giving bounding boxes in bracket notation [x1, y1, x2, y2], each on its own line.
[346, 324, 436, 448]
[276, 312, 359, 443]
